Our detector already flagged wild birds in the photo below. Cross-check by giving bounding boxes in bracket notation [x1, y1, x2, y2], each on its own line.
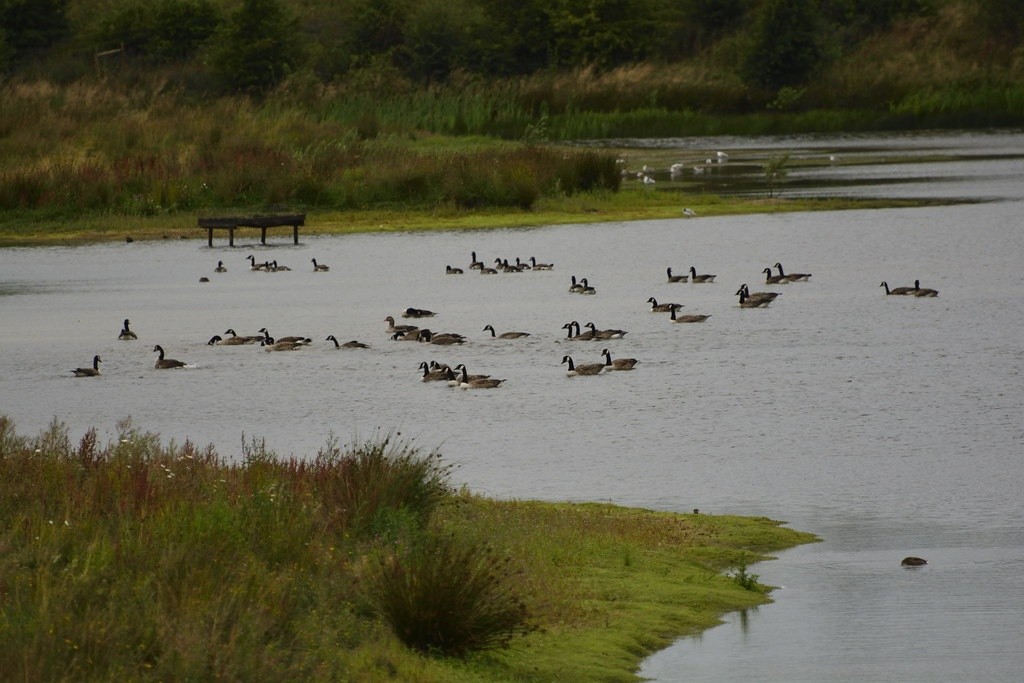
[310, 257, 331, 273]
[568, 275, 597, 295]
[117, 318, 138, 341]
[667, 266, 718, 284]
[878, 279, 942, 298]
[244, 254, 292, 273]
[381, 307, 469, 346]
[207, 328, 373, 352]
[198, 276, 210, 283]
[735, 284, 784, 308]
[417, 360, 508, 391]
[646, 297, 713, 325]
[613, 150, 731, 216]
[560, 321, 640, 379]
[482, 325, 532, 340]
[899, 557, 929, 572]
[445, 251, 554, 278]
[214, 260, 228, 273]
[152, 344, 188, 370]
[69, 355, 104, 377]
[761, 263, 812, 285]
